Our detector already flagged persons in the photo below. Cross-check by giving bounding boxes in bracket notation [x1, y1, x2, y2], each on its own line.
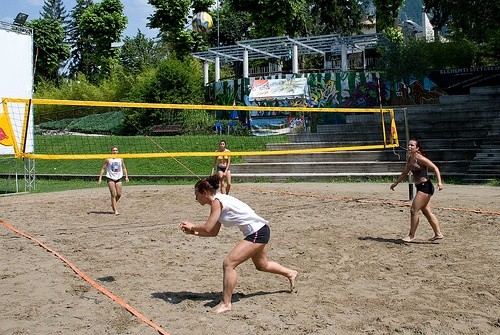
[211, 140, 231, 195]
[97, 147, 129, 216]
[179, 174, 298, 313]
[390, 139, 443, 242]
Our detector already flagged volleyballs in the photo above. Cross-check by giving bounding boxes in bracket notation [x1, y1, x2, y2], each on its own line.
[191, 12, 213, 34]
